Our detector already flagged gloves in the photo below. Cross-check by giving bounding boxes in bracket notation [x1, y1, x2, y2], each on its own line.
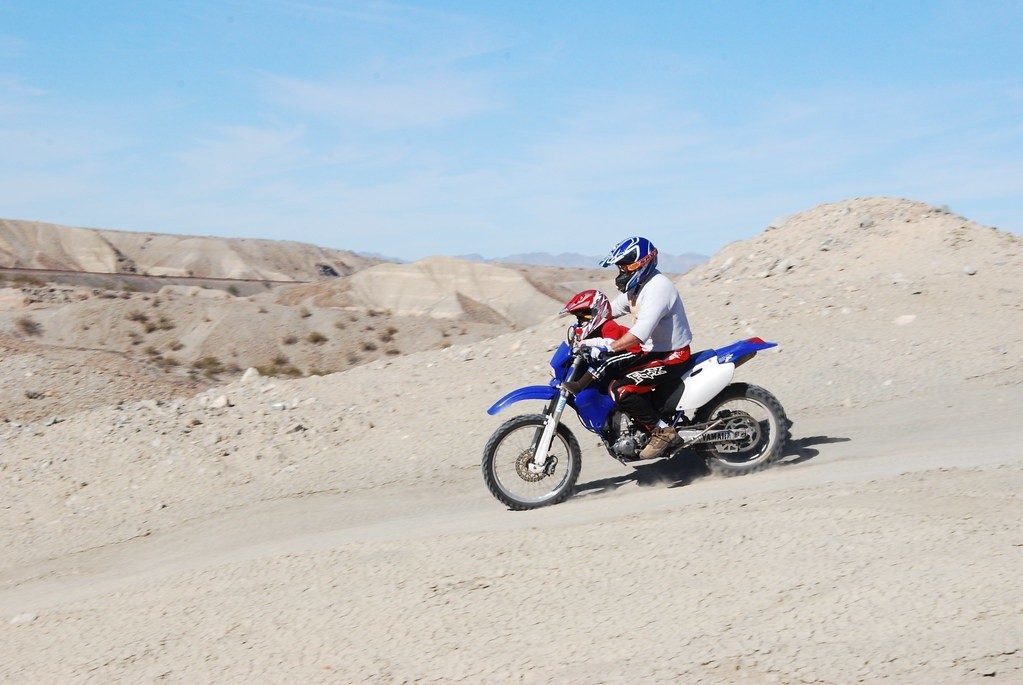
[589, 346, 613, 362]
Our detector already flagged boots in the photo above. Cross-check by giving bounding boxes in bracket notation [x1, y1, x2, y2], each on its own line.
[560, 367, 602, 398]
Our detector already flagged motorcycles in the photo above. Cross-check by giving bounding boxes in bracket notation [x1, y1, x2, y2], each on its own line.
[480, 321, 790, 512]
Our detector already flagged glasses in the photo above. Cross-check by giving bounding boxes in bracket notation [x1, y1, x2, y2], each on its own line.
[617, 265, 632, 277]
[577, 314, 586, 324]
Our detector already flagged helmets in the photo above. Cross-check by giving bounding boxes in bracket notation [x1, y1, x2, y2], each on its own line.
[597, 237, 658, 293]
[558, 290, 611, 342]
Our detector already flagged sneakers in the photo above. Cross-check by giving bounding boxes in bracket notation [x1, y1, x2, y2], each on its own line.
[639, 425, 684, 459]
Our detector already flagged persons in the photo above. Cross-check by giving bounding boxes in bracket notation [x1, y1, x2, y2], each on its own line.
[560, 290, 643, 398]
[589, 236, 692, 459]
